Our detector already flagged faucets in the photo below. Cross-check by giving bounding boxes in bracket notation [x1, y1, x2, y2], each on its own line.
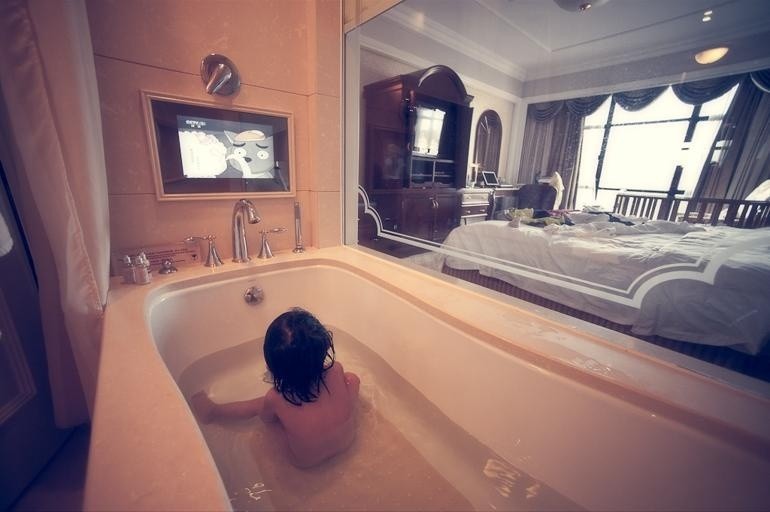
[230, 198, 262, 262]
[293, 201, 303, 249]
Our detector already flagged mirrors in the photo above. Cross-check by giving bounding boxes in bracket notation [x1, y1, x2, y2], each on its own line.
[411, 102, 451, 153]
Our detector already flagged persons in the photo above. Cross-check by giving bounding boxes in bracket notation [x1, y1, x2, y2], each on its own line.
[188, 304, 363, 469]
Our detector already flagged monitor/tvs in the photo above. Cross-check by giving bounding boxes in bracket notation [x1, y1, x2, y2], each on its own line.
[482, 171, 499, 186]
[411, 99, 454, 159]
[176, 112, 276, 179]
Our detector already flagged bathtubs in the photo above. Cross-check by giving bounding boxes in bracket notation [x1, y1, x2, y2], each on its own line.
[147, 259, 770, 511]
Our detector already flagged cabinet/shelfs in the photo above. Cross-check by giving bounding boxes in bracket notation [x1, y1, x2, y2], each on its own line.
[462, 192, 490, 225]
[353, 189, 460, 257]
[406, 155, 456, 189]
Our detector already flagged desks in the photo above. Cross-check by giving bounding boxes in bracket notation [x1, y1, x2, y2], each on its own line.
[489, 186, 519, 220]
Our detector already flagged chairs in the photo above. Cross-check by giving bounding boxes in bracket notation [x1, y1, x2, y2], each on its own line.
[493, 182, 559, 220]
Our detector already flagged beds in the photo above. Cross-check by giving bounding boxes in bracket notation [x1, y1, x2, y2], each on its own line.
[455, 210, 769, 358]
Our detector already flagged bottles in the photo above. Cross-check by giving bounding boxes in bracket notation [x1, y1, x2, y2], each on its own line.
[136, 255, 150, 284]
[139, 251, 153, 280]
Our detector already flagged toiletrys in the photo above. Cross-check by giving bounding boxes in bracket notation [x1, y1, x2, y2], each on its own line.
[131, 255, 150, 284]
[140, 251, 151, 274]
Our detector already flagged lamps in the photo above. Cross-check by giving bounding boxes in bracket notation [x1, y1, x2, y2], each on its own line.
[694, 46, 728, 65]
